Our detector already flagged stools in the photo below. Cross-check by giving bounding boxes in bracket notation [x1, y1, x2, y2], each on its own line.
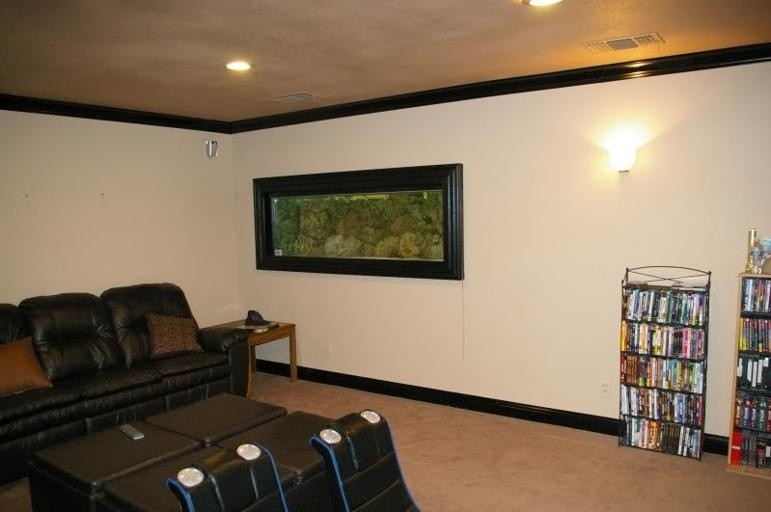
[28, 391, 361, 511]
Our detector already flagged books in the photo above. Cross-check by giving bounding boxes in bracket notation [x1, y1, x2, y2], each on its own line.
[621, 287, 707, 459]
[731, 277, 771, 470]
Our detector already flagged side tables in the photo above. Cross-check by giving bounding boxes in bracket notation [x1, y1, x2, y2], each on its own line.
[201, 317, 298, 398]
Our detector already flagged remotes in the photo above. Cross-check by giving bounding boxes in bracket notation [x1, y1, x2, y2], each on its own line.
[118, 422, 145, 441]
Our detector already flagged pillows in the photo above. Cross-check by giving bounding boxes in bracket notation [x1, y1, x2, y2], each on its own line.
[0, 336, 54, 398]
[144, 311, 206, 361]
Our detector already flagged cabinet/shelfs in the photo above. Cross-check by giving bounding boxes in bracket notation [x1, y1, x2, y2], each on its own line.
[618, 266, 711, 461]
[726, 271, 771, 480]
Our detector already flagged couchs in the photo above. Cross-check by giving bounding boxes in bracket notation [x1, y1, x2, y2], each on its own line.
[0, 282, 251, 485]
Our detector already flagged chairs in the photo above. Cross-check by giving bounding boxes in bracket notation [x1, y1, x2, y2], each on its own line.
[308, 407, 421, 512]
[165, 441, 290, 512]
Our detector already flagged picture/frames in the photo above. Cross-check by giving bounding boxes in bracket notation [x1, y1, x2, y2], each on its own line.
[252, 162, 464, 281]
